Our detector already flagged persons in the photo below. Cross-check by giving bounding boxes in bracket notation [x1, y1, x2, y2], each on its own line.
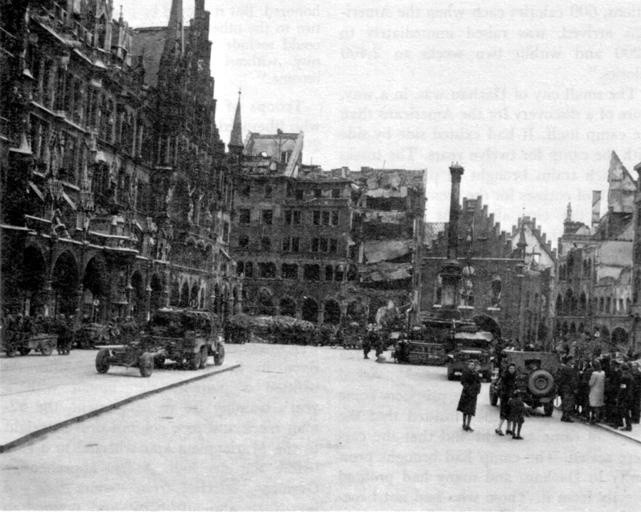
[555, 338, 641, 431]
[0, 309, 140, 357]
[456, 359, 483, 432]
[362, 327, 391, 359]
[495, 363, 517, 437]
[509, 373, 530, 439]
[499, 335, 554, 352]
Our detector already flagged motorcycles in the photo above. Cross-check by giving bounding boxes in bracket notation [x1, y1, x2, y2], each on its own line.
[6, 329, 58, 358]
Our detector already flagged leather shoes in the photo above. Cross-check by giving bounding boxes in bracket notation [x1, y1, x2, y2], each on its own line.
[463, 424, 474, 432]
[495, 429, 504, 436]
[506, 430, 523, 440]
[561, 416, 575, 422]
[580, 414, 639, 431]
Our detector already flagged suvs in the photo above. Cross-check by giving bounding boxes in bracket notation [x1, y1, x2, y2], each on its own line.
[446, 331, 498, 383]
[490, 347, 565, 417]
[143, 308, 227, 368]
